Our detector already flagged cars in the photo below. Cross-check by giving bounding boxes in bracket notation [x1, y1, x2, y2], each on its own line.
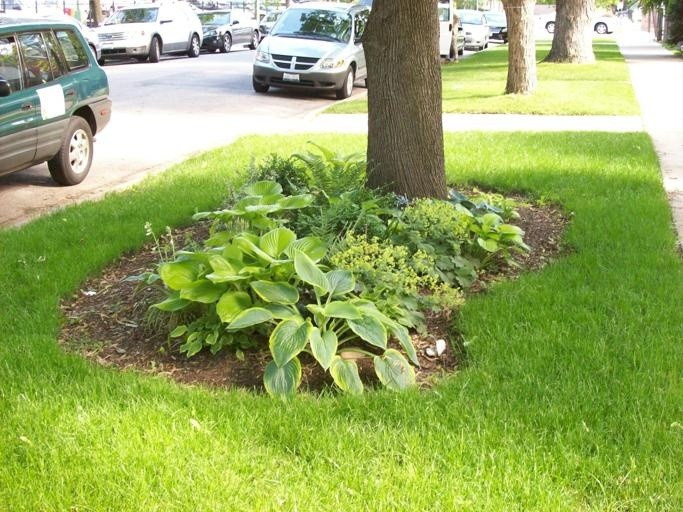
[0, 0, 259, 63]
[540, 10, 615, 34]
[249, 0, 508, 99]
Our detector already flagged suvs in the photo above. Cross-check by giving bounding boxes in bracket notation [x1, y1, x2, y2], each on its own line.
[0, 16, 111, 186]
